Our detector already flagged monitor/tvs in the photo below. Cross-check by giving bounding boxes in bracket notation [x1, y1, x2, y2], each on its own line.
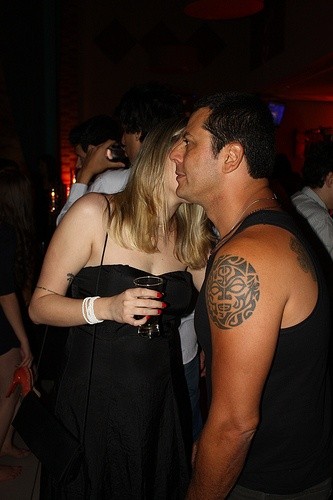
[265, 102, 287, 128]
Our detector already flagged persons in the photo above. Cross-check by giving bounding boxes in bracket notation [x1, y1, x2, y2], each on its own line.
[0, 166, 45, 481]
[56, 101, 184, 226]
[289, 138, 333, 258]
[12, 104, 215, 500]
[186, 91, 333, 500]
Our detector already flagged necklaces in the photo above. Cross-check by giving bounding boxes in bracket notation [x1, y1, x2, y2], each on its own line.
[210, 194, 281, 255]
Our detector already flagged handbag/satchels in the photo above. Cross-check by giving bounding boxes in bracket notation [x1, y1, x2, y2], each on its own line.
[12, 390, 86, 493]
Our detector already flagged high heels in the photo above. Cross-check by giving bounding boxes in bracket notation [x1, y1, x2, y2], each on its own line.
[5, 367, 31, 397]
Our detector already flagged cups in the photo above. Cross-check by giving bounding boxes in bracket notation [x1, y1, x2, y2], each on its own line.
[133, 277, 162, 338]
[105, 143, 128, 161]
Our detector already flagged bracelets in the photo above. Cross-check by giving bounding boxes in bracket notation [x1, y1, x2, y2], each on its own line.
[81, 296, 104, 326]
